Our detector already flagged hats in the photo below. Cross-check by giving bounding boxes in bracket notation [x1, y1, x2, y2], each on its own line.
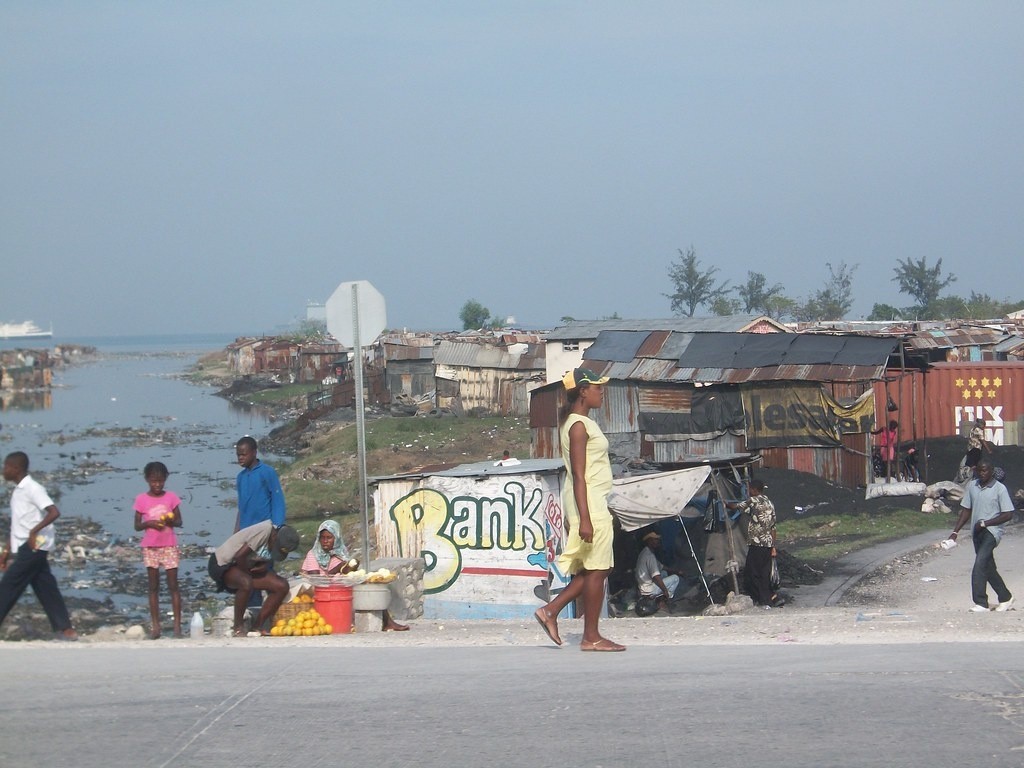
[906, 447, 919, 455]
[270, 526, 300, 563]
[562, 367, 611, 391]
[641, 531, 661, 542]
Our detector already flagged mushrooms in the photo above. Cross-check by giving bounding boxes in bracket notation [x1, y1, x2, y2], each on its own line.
[346, 558, 391, 577]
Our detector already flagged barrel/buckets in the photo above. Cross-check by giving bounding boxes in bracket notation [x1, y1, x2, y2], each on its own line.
[313, 587, 351, 635]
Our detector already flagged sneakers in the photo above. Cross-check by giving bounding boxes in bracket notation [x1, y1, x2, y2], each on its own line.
[968, 604, 991, 613]
[995, 594, 1016, 611]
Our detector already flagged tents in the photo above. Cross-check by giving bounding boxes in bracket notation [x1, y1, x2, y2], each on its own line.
[608, 465, 741, 606]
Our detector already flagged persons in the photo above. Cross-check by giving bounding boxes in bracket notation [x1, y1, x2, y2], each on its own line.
[946, 458, 1015, 614]
[722, 479, 783, 609]
[871, 420, 898, 484]
[0, 451, 78, 641]
[294, 520, 410, 632]
[232, 438, 286, 607]
[534, 369, 626, 652]
[634, 531, 679, 613]
[964, 418, 993, 481]
[208, 519, 300, 637]
[903, 449, 919, 483]
[133, 461, 182, 639]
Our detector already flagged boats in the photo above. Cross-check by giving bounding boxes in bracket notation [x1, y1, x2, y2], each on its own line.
[0, 320, 52, 340]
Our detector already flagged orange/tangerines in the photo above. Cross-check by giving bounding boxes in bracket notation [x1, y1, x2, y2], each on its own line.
[157, 512, 174, 526]
[271, 594, 334, 635]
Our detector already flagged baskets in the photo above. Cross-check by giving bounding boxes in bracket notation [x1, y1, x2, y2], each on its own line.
[268, 602, 314, 631]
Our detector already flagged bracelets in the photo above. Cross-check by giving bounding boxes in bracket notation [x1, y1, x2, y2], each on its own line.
[952, 532, 957, 535]
[980, 521, 986, 528]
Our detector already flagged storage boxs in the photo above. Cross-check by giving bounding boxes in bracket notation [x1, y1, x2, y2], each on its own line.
[271, 602, 314, 630]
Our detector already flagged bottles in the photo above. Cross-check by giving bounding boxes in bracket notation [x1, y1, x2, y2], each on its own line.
[189, 611, 204, 639]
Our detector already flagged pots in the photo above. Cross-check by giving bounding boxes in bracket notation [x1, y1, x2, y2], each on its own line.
[351, 582, 391, 611]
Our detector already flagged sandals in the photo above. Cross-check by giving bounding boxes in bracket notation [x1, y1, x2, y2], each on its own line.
[578, 637, 626, 652]
[533, 607, 565, 645]
